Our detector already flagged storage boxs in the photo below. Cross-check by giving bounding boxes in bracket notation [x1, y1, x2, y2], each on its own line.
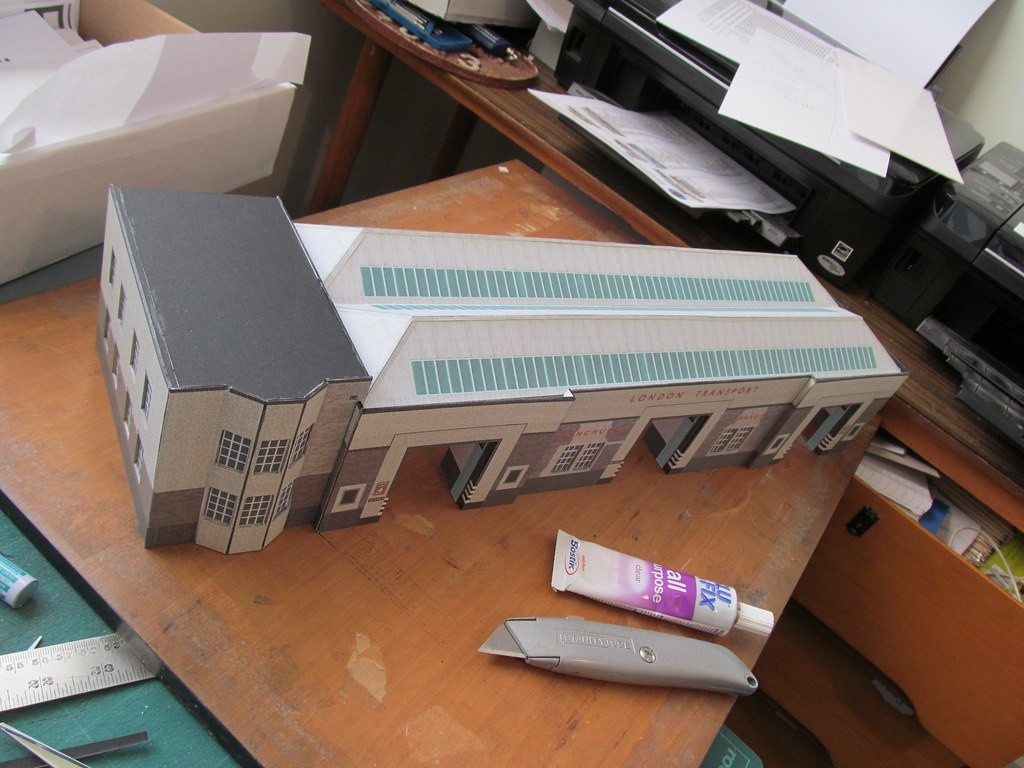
[0, 0, 301, 295]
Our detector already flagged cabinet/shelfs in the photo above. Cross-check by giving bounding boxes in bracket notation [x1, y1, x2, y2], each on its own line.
[734, 401, 1024, 768]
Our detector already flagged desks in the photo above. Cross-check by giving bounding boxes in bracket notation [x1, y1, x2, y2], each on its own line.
[307, 0, 1023, 499]
[0, 161, 865, 768]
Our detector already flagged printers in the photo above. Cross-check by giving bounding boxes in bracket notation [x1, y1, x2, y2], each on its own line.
[553, 0, 987, 292]
[864, 139, 1024, 456]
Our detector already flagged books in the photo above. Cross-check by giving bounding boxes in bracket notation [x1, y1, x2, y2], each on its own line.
[856, 439, 940, 521]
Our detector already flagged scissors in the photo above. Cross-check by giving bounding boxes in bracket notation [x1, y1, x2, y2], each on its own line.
[0, 720, 92, 768]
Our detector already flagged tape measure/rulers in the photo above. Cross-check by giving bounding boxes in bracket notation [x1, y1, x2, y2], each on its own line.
[0, 633, 159, 714]
